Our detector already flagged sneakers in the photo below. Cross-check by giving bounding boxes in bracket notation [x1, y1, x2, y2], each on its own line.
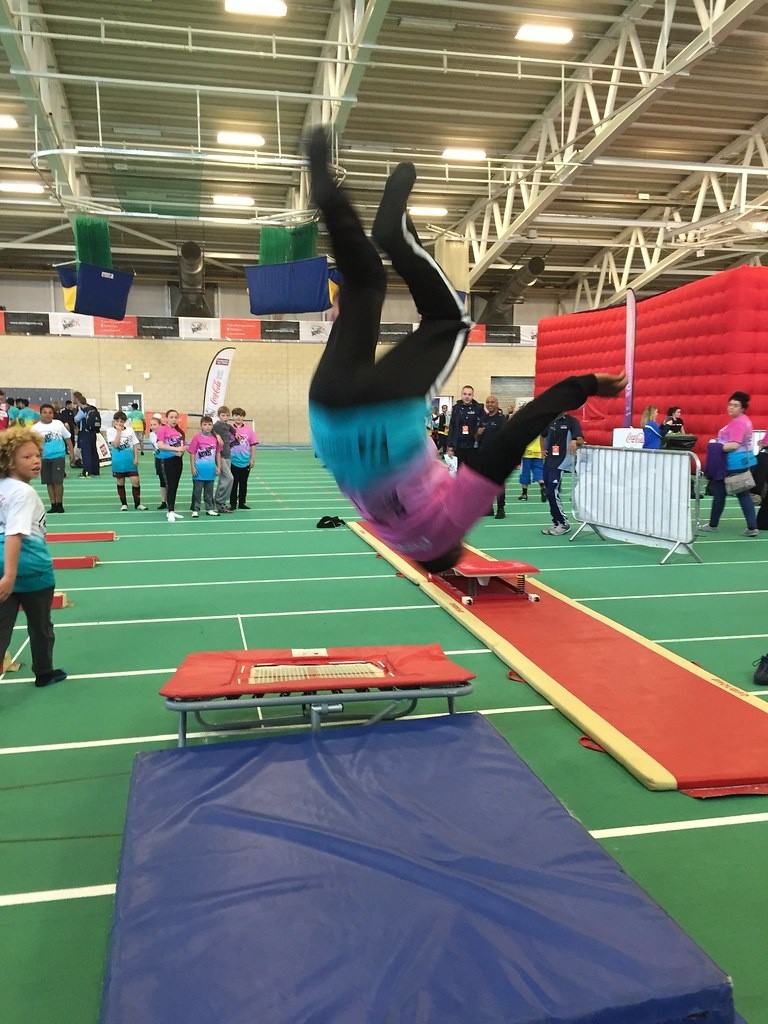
[541, 525, 558, 535]
[549, 524, 570, 536]
[121, 505, 128, 511]
[137, 505, 147, 510]
[698, 523, 718, 532]
[741, 528, 758, 536]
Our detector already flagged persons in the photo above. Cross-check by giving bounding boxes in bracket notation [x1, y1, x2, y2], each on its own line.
[187, 405, 259, 517]
[61, 400, 79, 454]
[149, 409, 187, 522]
[106, 411, 149, 510]
[518, 433, 546, 502]
[541, 412, 584, 536]
[640, 405, 673, 449]
[305, 122, 630, 573]
[475, 395, 509, 519]
[697, 392, 759, 535]
[448, 385, 488, 465]
[0, 425, 67, 687]
[71, 391, 100, 478]
[0, 389, 41, 431]
[126, 403, 146, 455]
[430, 405, 449, 458]
[506, 405, 514, 418]
[30, 403, 75, 513]
[52, 400, 63, 420]
[659, 407, 686, 434]
[756, 429, 768, 530]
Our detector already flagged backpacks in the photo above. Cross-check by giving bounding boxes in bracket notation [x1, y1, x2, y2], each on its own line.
[83, 407, 101, 432]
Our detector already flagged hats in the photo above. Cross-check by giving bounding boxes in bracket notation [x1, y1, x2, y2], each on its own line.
[153, 413, 162, 419]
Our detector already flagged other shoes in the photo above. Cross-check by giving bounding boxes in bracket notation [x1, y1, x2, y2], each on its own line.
[494, 508, 506, 519]
[218, 506, 233, 513]
[541, 487, 546, 502]
[167, 512, 184, 518]
[691, 493, 703, 499]
[192, 511, 199, 517]
[168, 512, 176, 522]
[206, 510, 220, 516]
[486, 507, 494, 516]
[45, 668, 67, 685]
[518, 493, 527, 501]
[78, 472, 94, 478]
[238, 504, 251, 509]
[752, 655, 768, 685]
[157, 501, 166, 510]
[229, 506, 236, 510]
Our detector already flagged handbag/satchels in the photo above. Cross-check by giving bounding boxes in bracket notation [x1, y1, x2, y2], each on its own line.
[444, 454, 458, 478]
[724, 471, 756, 494]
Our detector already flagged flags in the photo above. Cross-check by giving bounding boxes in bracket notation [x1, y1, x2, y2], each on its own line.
[203, 347, 236, 425]
